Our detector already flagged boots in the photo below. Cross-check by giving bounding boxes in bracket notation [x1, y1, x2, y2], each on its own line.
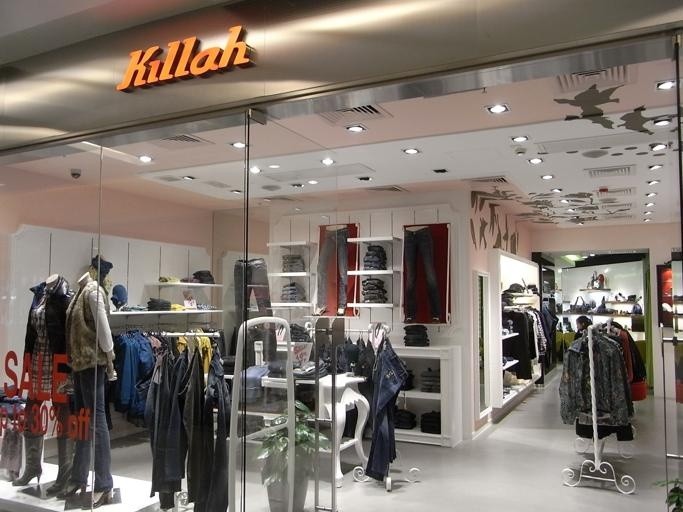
[11, 431, 43, 487]
[45, 434, 76, 493]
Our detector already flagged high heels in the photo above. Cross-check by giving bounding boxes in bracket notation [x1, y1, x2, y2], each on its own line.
[55, 479, 86, 500]
[80, 489, 114, 509]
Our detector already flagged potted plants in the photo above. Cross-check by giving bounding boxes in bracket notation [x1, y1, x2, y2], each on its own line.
[253, 399, 330, 512]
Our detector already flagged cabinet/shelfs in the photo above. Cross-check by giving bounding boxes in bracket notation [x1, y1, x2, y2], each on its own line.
[487, 248, 541, 409]
[254, 340, 463, 449]
[345, 236, 401, 308]
[266, 240, 317, 308]
[109, 281, 224, 316]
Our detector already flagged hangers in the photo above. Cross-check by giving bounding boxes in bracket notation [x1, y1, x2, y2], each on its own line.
[114, 321, 164, 337]
[582, 325, 606, 342]
[163, 331, 220, 353]
[344, 329, 371, 340]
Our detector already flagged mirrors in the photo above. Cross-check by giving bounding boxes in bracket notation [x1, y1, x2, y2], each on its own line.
[473, 270, 492, 421]
[211, 205, 270, 375]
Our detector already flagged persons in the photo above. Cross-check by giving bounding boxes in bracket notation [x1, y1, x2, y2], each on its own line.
[56, 271, 116, 510]
[405, 226, 440, 321]
[312, 225, 349, 315]
[574, 316, 592, 340]
[12, 273, 75, 494]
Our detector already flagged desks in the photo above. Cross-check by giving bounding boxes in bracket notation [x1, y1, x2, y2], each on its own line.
[222, 374, 368, 489]
